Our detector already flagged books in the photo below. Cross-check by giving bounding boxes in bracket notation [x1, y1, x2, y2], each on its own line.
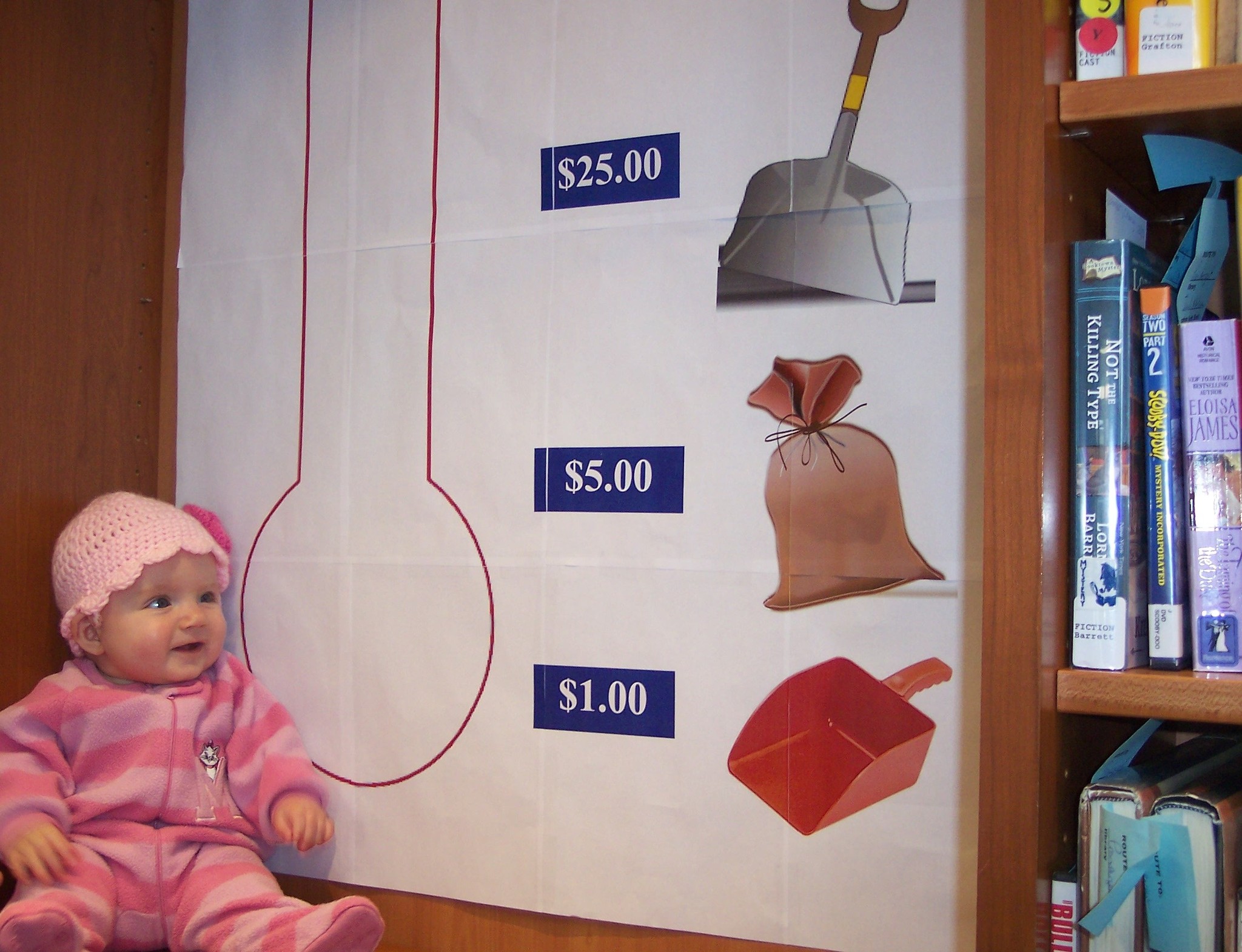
[1051, 734, 1242, 952]
[1075, 0, 1242, 81]
[1073, 237, 1242, 673]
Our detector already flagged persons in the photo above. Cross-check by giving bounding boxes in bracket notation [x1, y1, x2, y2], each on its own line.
[1, 491, 385, 952]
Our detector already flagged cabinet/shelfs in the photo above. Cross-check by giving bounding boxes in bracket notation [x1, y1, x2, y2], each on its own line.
[981, 0, 1242, 951]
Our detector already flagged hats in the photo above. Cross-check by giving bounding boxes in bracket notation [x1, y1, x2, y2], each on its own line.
[50, 491, 232, 658]
